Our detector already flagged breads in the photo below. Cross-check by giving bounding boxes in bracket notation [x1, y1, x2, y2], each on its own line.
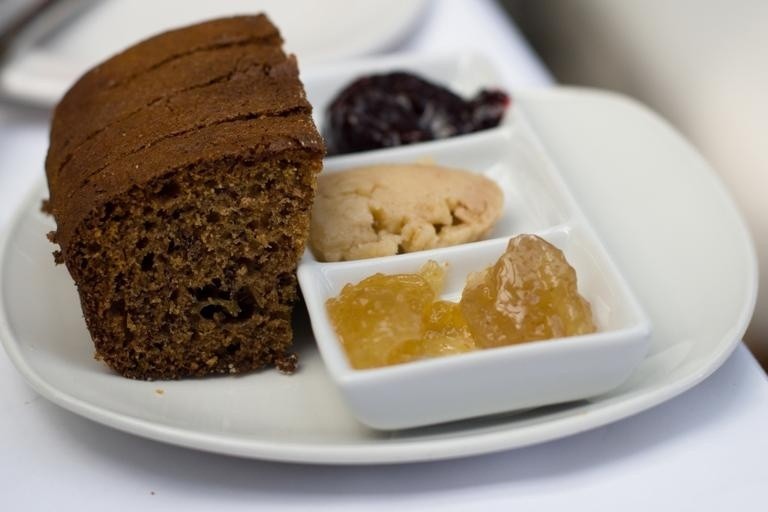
[45, 12, 326, 377]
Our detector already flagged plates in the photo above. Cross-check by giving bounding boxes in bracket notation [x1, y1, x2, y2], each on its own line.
[0, 69, 760, 469]
[280, 37, 654, 438]
[0, 0, 434, 107]
